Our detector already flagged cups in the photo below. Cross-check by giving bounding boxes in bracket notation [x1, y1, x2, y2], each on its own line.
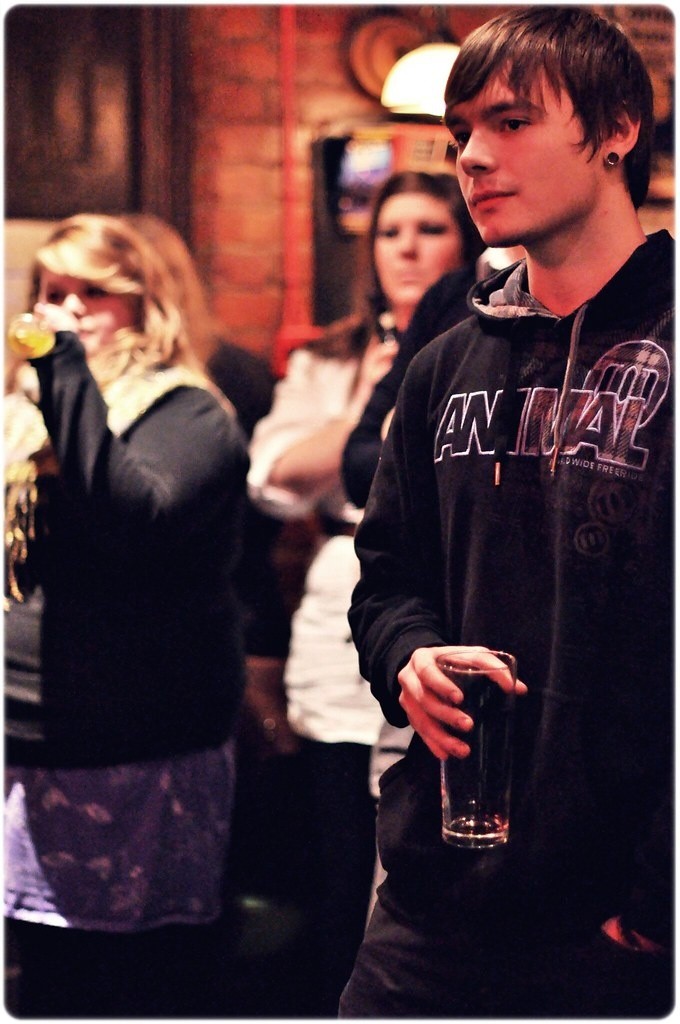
[6, 314, 54, 356]
[438, 653, 516, 848]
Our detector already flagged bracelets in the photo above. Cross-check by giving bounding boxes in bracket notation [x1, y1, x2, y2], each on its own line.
[620, 918, 647, 953]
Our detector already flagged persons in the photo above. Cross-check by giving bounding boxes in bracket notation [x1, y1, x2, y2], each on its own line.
[340, 6, 675, 1018]
[0, 214, 324, 1018]
[246, 172, 485, 1018]
